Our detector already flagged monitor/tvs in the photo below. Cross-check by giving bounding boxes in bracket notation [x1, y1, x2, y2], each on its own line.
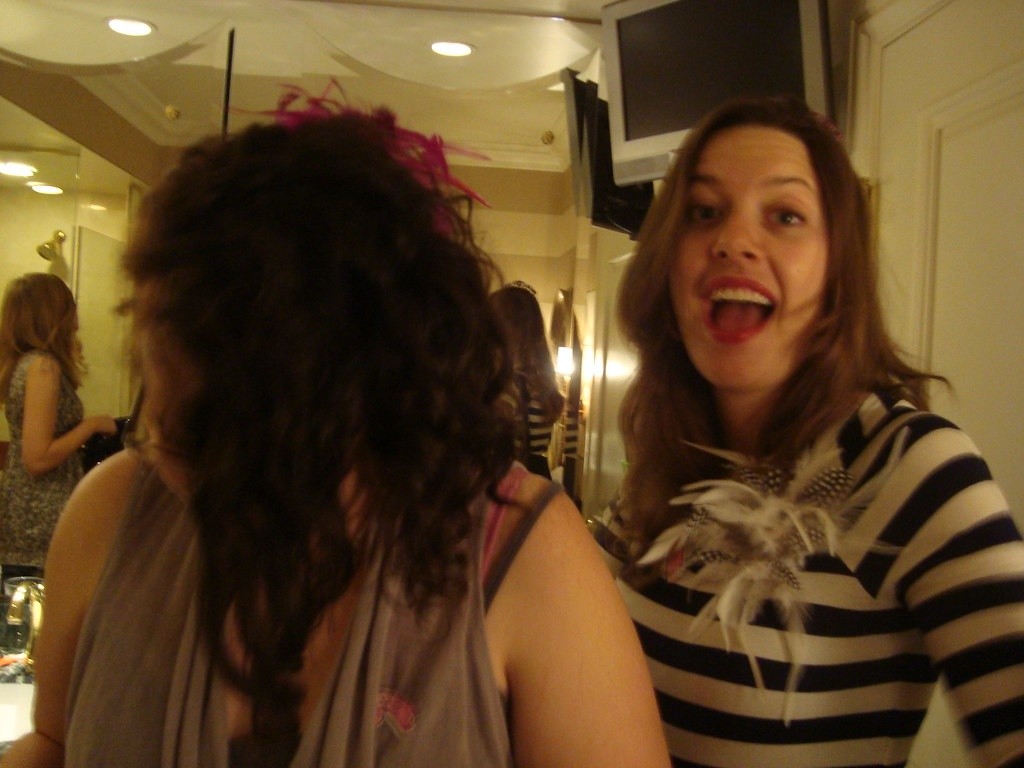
[561, 0, 833, 240]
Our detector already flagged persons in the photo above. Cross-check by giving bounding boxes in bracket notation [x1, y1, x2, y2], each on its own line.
[582, 92, 1024, 767]
[0, 272, 117, 567]
[562, 370, 580, 496]
[487, 282, 564, 483]
[0, 112, 672, 768]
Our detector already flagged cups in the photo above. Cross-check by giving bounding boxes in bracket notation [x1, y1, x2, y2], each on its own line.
[0, 575, 44, 683]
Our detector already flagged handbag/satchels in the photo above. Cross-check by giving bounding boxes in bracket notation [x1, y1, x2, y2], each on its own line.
[84, 417, 128, 469]
